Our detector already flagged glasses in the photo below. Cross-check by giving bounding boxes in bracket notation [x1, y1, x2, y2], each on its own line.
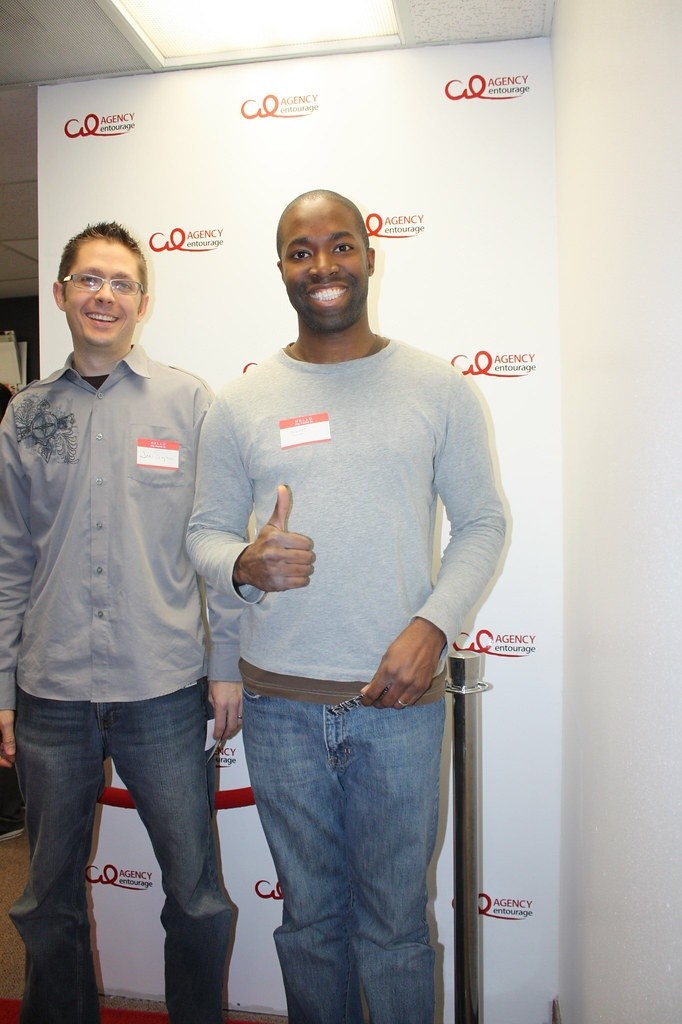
[61, 274, 144, 295]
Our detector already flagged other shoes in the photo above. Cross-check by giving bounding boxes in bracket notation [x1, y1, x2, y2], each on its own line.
[0, 820, 25, 841]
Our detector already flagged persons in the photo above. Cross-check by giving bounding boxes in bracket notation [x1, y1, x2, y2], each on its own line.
[0, 221, 244, 1024]
[185, 188, 507, 1023]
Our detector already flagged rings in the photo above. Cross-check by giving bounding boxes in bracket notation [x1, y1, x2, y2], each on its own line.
[398, 699, 409, 706]
[238, 715, 242, 719]
